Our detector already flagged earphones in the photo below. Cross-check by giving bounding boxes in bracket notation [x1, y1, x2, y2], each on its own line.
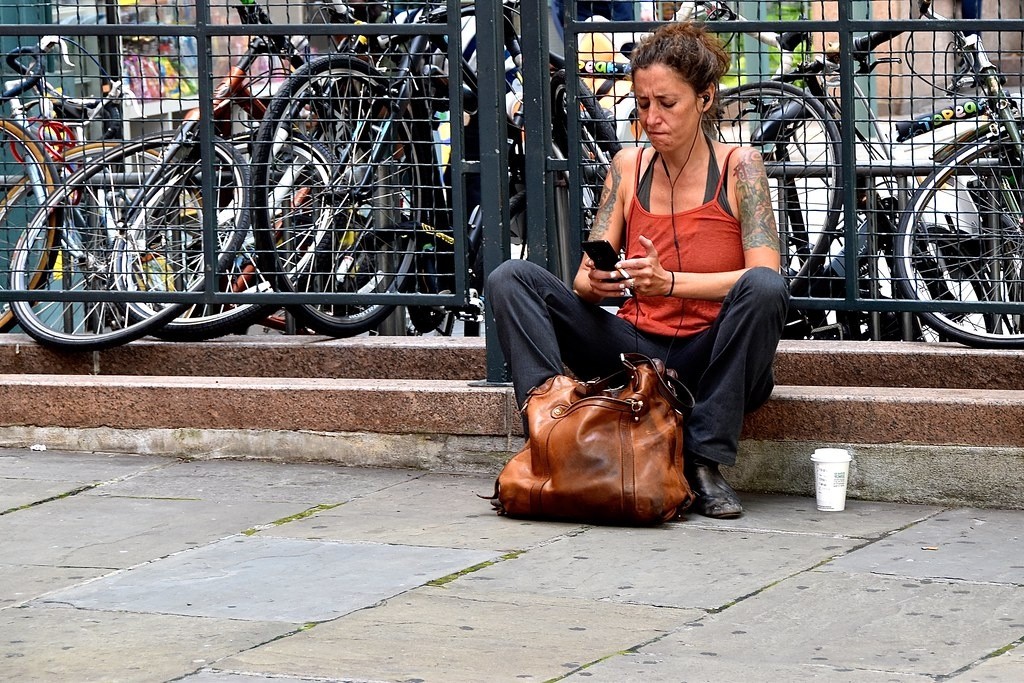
[702, 95, 710, 104]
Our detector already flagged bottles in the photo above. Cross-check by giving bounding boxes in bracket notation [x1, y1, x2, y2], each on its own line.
[810, 448, 852, 512]
[831, 194, 897, 278]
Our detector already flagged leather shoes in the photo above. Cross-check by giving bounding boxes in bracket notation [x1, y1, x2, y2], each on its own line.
[684, 461, 742, 518]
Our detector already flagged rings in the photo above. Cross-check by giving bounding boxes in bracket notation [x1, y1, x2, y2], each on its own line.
[629, 278, 635, 289]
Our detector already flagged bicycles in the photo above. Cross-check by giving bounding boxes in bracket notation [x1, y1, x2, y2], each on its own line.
[0, 1, 1023, 351]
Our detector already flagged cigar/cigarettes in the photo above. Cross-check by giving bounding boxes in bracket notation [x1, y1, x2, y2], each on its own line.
[617, 269, 630, 279]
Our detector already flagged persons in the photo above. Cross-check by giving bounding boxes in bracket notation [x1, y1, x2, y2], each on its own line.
[486, 21, 790, 519]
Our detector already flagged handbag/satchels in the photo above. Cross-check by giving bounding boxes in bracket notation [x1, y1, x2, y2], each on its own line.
[476, 353, 696, 526]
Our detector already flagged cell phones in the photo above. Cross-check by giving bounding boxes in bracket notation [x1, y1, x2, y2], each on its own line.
[581, 239, 626, 285]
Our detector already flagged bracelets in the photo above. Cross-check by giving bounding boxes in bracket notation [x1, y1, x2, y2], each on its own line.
[665, 271, 674, 298]
